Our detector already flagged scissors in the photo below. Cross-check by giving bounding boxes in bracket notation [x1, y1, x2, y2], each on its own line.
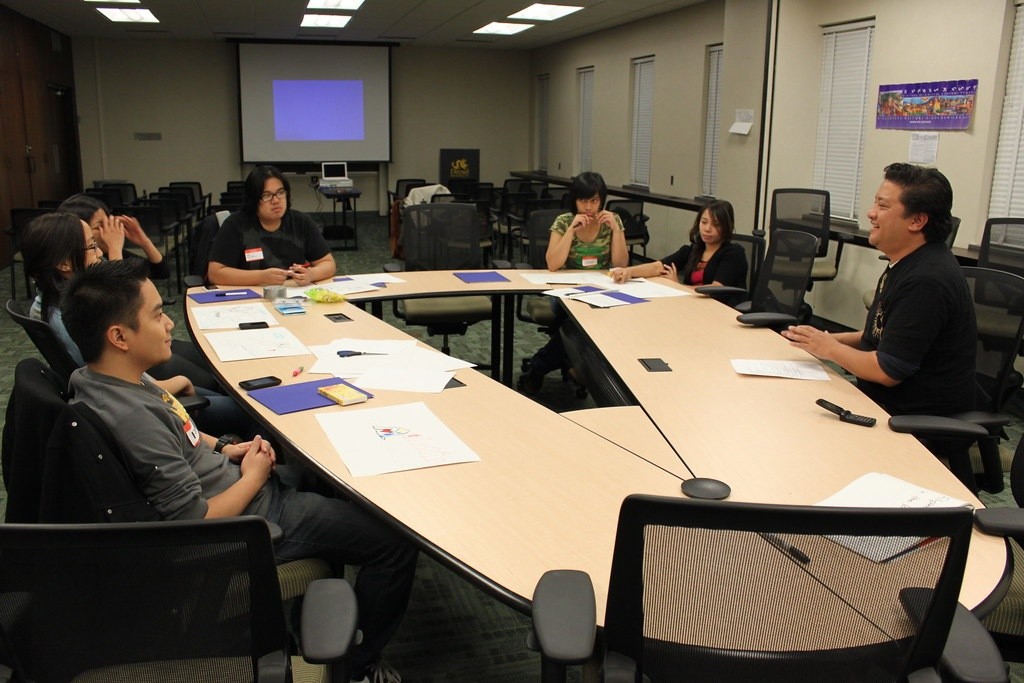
[337, 351, 389, 357]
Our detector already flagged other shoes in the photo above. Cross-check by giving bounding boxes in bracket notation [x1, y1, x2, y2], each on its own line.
[525, 352, 547, 393]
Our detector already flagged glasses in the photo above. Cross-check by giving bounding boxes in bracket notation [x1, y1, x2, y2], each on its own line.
[259, 189, 287, 202]
[83, 242, 98, 250]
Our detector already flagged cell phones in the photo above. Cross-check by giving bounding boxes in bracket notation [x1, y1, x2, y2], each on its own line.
[203, 284, 220, 292]
[816, 399, 876, 427]
[239, 376, 282, 391]
[239, 322, 268, 330]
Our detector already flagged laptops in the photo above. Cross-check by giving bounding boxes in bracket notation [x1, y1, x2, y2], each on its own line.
[322, 162, 349, 182]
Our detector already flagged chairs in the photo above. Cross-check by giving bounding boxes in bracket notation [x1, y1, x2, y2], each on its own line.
[1, 169, 1024, 683]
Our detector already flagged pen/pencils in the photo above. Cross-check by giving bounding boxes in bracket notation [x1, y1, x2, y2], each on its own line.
[216, 292, 247, 296]
[573, 216, 595, 228]
[288, 262, 310, 272]
[758, 532, 811, 565]
[293, 367, 303, 376]
[625, 280, 645, 283]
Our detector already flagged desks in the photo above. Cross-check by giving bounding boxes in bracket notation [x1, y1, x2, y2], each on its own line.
[183, 265, 1017, 659]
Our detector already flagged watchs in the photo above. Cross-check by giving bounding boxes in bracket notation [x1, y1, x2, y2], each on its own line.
[213, 433, 244, 454]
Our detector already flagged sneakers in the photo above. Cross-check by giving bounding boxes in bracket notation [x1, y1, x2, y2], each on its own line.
[364, 655, 401, 683]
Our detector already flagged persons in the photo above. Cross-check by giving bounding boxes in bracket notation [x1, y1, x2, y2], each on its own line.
[56, 195, 223, 389]
[60, 261, 419, 682]
[523, 172, 631, 393]
[24, 214, 286, 466]
[608, 200, 748, 296]
[781, 162, 978, 463]
[207, 163, 336, 285]
